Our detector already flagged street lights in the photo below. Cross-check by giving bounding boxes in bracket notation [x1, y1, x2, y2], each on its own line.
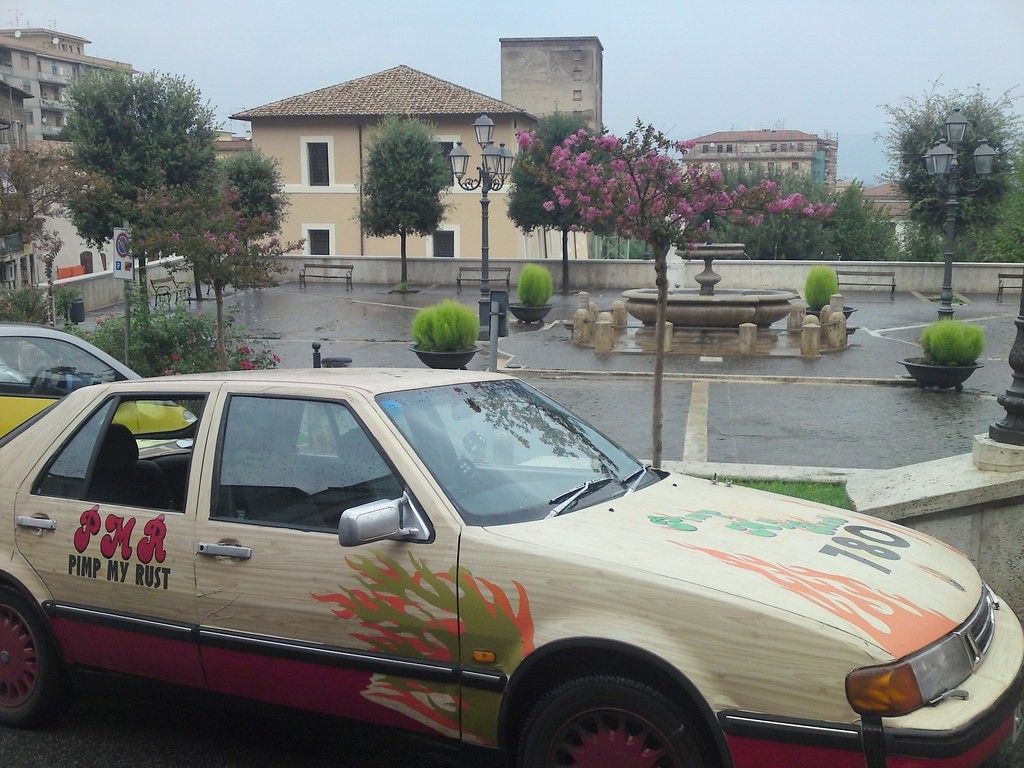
[451, 114, 513, 341]
[921, 110, 1000, 320]
[43, 253, 57, 326]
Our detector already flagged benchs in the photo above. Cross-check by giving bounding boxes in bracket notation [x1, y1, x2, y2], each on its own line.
[205, 272, 226, 295]
[148, 275, 191, 310]
[454, 266, 511, 295]
[297, 263, 354, 292]
[833, 270, 898, 301]
[83, 422, 190, 514]
[996, 273, 1024, 303]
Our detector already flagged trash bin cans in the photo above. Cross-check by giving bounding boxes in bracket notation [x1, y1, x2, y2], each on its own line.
[65, 298, 85, 322]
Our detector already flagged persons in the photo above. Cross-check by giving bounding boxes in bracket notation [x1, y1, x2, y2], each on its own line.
[336, 427, 385, 508]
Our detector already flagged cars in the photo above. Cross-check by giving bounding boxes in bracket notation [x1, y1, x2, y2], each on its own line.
[0, 366, 1024, 768]
[0, 322, 200, 449]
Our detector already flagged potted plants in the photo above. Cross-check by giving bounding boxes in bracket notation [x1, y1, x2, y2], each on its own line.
[406, 298, 484, 370]
[507, 261, 553, 324]
[894, 316, 989, 389]
[800, 262, 859, 322]
